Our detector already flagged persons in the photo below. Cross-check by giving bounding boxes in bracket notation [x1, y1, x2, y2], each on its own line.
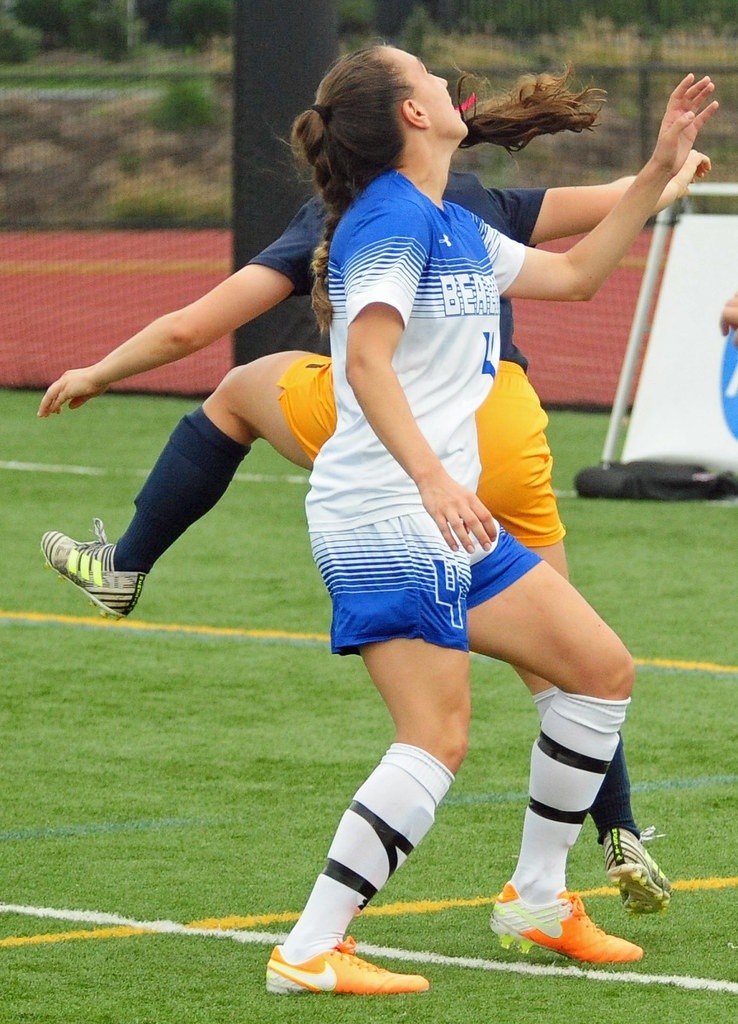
[264, 44, 724, 1002]
[719, 295, 738, 346]
[35, 71, 712, 914]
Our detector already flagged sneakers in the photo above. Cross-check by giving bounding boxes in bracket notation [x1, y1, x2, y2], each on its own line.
[604, 825, 674, 915]
[266, 937, 429, 993]
[490, 882, 644, 962]
[40, 517, 147, 621]
